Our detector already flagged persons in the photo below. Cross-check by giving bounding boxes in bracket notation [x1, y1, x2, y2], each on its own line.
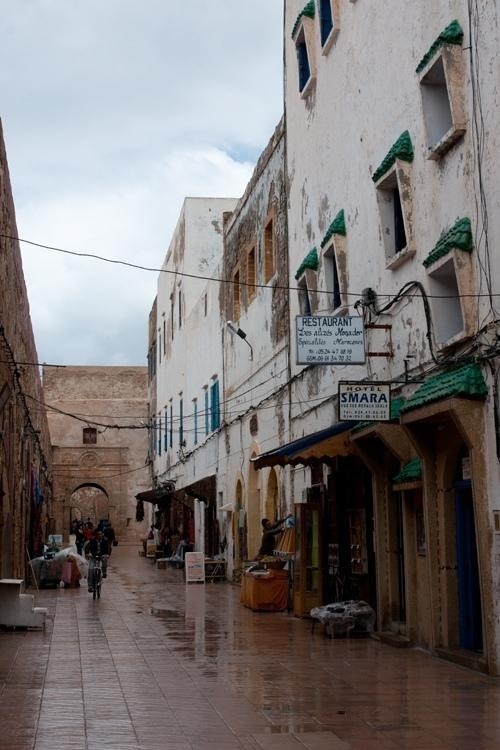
[72, 514, 118, 593]
[258, 512, 293, 556]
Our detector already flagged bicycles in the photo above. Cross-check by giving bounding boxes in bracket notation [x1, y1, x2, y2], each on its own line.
[85, 552, 105, 601]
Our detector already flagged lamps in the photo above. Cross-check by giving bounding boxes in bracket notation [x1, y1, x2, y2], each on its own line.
[225, 319, 253, 361]
